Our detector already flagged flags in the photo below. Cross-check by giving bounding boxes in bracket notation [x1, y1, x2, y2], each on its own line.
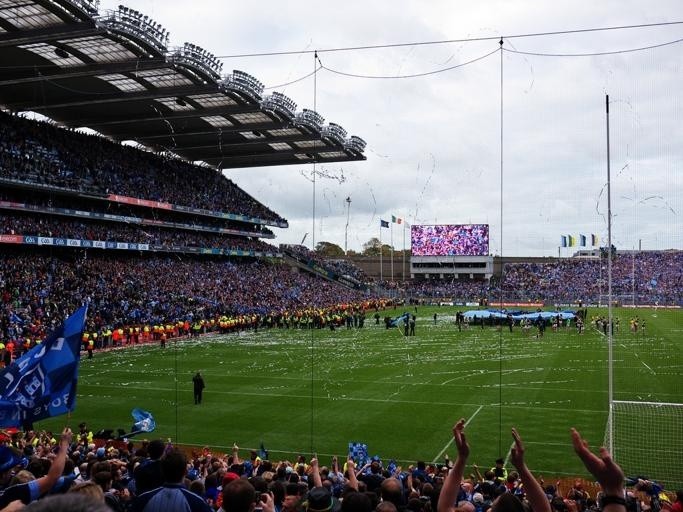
[405, 222, 409, 229]
[119, 408, 155, 441]
[0, 301, 87, 429]
[392, 216, 401, 224]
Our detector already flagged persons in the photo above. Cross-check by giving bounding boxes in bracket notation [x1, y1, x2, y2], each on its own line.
[192, 373, 204, 404]
[410, 225, 488, 256]
[1, 419, 683, 511]
[394, 251, 683, 335]
[1, 110, 395, 366]
